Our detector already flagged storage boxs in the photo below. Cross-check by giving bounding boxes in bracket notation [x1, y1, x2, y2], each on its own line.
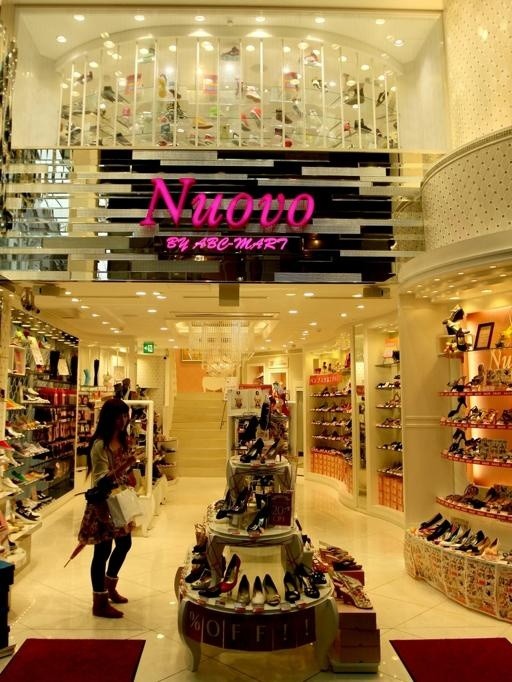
[332, 604, 378, 631]
[325, 644, 383, 665]
[332, 569, 365, 589]
[332, 627, 382, 650]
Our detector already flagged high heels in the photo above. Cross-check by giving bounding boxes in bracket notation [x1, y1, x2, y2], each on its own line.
[301, 560, 327, 585]
[445, 484, 480, 504]
[446, 323, 471, 336]
[420, 514, 446, 536]
[448, 397, 468, 418]
[461, 531, 486, 552]
[440, 527, 464, 547]
[340, 583, 374, 611]
[427, 520, 451, 541]
[319, 547, 364, 570]
[467, 537, 492, 557]
[247, 500, 273, 534]
[198, 583, 224, 597]
[252, 577, 266, 608]
[442, 304, 464, 325]
[446, 375, 467, 386]
[294, 563, 320, 599]
[263, 574, 281, 607]
[215, 490, 232, 520]
[449, 528, 474, 547]
[255, 491, 269, 509]
[260, 438, 286, 463]
[220, 555, 241, 594]
[237, 575, 250, 609]
[459, 407, 501, 425]
[456, 329, 467, 353]
[333, 574, 368, 598]
[240, 438, 265, 464]
[283, 571, 300, 603]
[434, 522, 458, 544]
[471, 363, 486, 386]
[474, 488, 497, 510]
[448, 429, 467, 452]
[228, 488, 251, 514]
[419, 510, 443, 532]
[485, 538, 503, 556]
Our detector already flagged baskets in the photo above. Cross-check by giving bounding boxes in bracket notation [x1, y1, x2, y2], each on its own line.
[156, 461, 178, 480]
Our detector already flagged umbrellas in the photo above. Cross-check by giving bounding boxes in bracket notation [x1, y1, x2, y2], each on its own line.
[63, 543, 84, 568]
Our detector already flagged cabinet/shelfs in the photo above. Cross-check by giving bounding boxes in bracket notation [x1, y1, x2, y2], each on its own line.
[155, 438, 177, 486]
[401, 295, 512, 623]
[300, 350, 366, 513]
[0, 302, 79, 581]
[72, 399, 156, 536]
[366, 331, 404, 525]
[175, 404, 334, 674]
[78, 385, 111, 465]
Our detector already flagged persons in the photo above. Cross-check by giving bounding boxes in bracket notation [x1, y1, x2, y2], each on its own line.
[254, 391, 261, 408]
[78, 399, 136, 618]
[235, 391, 243, 408]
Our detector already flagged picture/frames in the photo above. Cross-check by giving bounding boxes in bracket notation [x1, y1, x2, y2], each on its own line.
[180, 349, 203, 363]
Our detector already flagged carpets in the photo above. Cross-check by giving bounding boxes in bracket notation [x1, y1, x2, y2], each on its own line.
[388, 637, 511, 682]
[1, 637, 147, 682]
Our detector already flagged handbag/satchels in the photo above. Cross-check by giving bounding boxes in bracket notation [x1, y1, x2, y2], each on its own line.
[106, 481, 145, 530]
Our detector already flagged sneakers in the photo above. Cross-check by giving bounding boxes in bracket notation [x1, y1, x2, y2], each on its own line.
[204, 134, 216, 143]
[28, 491, 52, 503]
[157, 75, 167, 99]
[210, 107, 223, 117]
[375, 89, 390, 104]
[61, 104, 72, 115]
[122, 106, 131, 119]
[166, 102, 185, 115]
[313, 382, 354, 398]
[189, 134, 205, 146]
[91, 109, 109, 121]
[276, 109, 292, 122]
[115, 132, 132, 146]
[159, 134, 177, 145]
[273, 128, 291, 140]
[159, 445, 176, 456]
[192, 568, 213, 588]
[220, 46, 240, 59]
[375, 393, 404, 409]
[376, 460, 404, 476]
[343, 121, 351, 131]
[168, 82, 182, 100]
[73, 101, 87, 114]
[377, 128, 382, 136]
[313, 430, 343, 442]
[5, 414, 43, 439]
[1, 471, 50, 488]
[249, 132, 267, 147]
[9, 440, 51, 457]
[291, 100, 304, 115]
[246, 86, 263, 101]
[297, 51, 319, 62]
[372, 376, 402, 390]
[23, 387, 50, 403]
[312, 401, 352, 413]
[377, 439, 404, 453]
[312, 416, 351, 428]
[5, 399, 27, 410]
[251, 108, 264, 122]
[103, 84, 121, 99]
[142, 47, 157, 61]
[13, 505, 39, 524]
[192, 537, 206, 554]
[192, 116, 214, 130]
[65, 122, 81, 135]
[65, 135, 80, 144]
[75, 72, 93, 83]
[312, 77, 328, 91]
[230, 132, 250, 147]
[376, 416, 403, 429]
[239, 113, 247, 126]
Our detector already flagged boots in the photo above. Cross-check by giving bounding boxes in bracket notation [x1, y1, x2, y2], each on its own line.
[82, 369, 91, 386]
[113, 378, 132, 400]
[344, 84, 365, 105]
[353, 118, 371, 131]
[92, 359, 101, 387]
[103, 572, 129, 604]
[90, 589, 125, 621]
